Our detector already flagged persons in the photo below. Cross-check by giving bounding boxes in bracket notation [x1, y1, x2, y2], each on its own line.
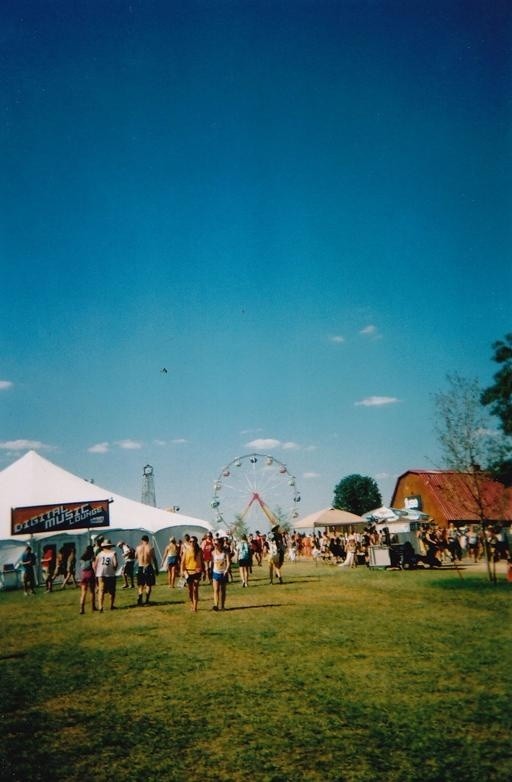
[78, 533, 159, 614]
[19, 545, 77, 595]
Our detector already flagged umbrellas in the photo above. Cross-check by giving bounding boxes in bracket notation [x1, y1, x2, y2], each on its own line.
[293, 508, 365, 529]
[362, 505, 429, 522]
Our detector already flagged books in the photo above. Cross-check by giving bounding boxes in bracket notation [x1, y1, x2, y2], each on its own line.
[164, 524, 287, 609]
[288, 523, 512, 581]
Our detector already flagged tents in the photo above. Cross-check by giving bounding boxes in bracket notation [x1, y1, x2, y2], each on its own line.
[0, 451, 216, 588]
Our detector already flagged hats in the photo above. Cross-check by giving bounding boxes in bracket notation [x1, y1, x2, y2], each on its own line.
[272, 524, 280, 532]
[90, 533, 104, 546]
[101, 539, 116, 548]
